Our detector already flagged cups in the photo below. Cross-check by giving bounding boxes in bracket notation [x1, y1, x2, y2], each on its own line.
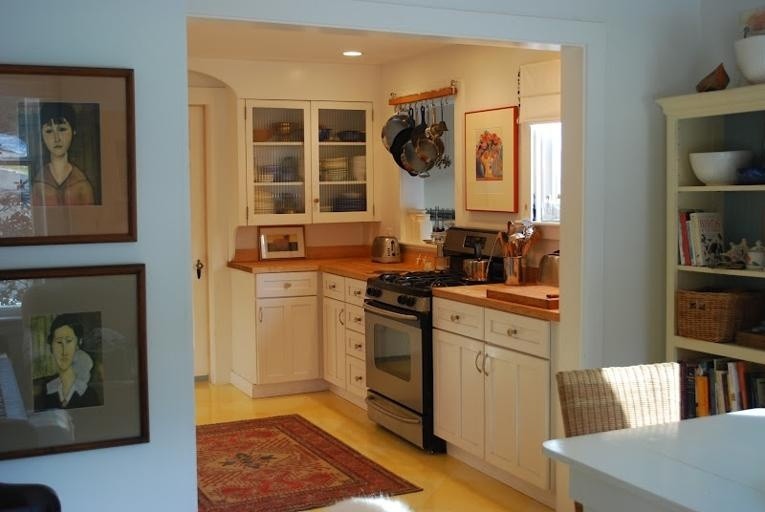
[741, 241, 765, 269]
[503, 256, 526, 286]
[416, 252, 451, 270]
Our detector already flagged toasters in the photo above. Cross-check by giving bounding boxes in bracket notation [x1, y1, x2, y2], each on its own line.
[371, 236, 402, 264]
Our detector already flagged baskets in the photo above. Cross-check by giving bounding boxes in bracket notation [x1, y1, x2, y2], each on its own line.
[676, 285, 765, 344]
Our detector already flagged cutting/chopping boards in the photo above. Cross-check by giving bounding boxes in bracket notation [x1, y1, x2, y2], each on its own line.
[486, 285, 560, 309]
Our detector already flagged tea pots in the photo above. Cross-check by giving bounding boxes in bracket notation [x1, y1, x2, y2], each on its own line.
[535, 249, 560, 288]
[461, 242, 493, 283]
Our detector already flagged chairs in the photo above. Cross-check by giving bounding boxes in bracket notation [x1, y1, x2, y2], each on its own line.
[1, 482, 61, 512]
[555, 363, 681, 512]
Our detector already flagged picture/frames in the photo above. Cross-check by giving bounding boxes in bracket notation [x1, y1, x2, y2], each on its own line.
[0, 263, 149, 461]
[257, 225, 306, 260]
[0, 64, 137, 245]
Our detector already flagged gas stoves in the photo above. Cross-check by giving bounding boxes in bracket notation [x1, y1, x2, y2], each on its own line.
[364, 268, 502, 314]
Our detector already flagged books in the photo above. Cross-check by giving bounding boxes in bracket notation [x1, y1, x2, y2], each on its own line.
[678, 204, 727, 268]
[678, 354, 765, 421]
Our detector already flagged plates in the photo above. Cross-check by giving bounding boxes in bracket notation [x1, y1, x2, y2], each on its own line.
[332, 197, 366, 211]
[340, 192, 363, 197]
[255, 174, 275, 183]
[255, 191, 278, 214]
[320, 169, 349, 181]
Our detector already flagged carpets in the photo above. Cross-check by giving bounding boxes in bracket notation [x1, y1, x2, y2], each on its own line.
[197, 414, 423, 512]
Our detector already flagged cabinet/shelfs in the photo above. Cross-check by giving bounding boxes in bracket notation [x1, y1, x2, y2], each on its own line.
[321, 272, 368, 400]
[656, 84, 764, 417]
[254, 270, 321, 386]
[431, 296, 552, 493]
[245, 98, 374, 227]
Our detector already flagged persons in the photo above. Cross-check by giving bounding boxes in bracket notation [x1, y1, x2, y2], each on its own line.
[34, 313, 99, 411]
[31, 101, 94, 206]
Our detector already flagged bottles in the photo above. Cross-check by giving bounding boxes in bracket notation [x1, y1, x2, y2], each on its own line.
[542, 195, 553, 222]
[280, 193, 297, 214]
[553, 194, 561, 221]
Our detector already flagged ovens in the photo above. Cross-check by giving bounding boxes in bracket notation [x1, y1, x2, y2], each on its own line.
[363, 299, 448, 453]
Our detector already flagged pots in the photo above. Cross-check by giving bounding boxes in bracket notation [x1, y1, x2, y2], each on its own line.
[381, 107, 444, 180]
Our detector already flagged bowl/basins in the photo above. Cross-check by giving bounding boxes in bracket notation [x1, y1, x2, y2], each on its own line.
[337, 130, 357, 141]
[252, 128, 274, 141]
[689, 150, 754, 187]
[696, 62, 729, 92]
[320, 157, 350, 170]
[319, 128, 332, 141]
[733, 34, 765, 85]
[352, 155, 366, 180]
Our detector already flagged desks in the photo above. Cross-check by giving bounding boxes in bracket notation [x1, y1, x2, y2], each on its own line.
[542, 409, 765, 512]
